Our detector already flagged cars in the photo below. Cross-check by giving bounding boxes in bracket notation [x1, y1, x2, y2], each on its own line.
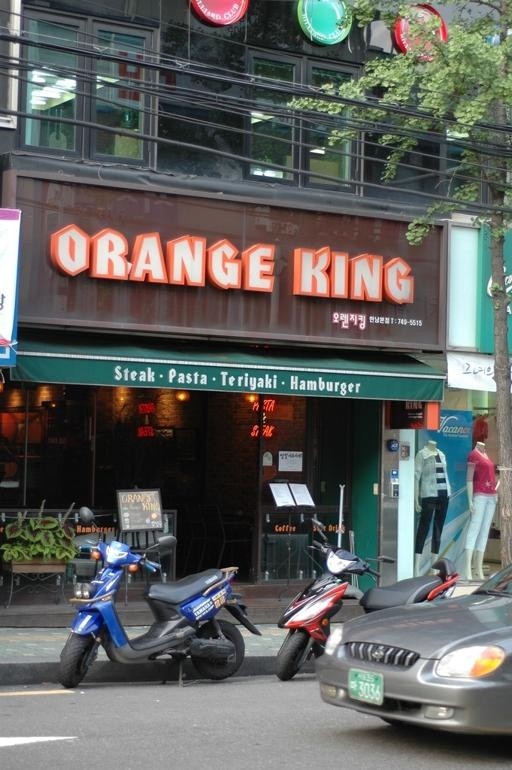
[315, 564, 512, 734]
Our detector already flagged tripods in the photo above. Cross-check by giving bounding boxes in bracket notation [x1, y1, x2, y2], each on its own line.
[278, 514, 301, 603]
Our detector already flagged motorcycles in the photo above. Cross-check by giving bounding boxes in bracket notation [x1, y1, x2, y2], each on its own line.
[276, 519, 460, 681]
[58, 507, 261, 688]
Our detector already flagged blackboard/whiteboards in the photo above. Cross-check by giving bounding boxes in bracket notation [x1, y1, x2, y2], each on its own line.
[116, 488, 165, 532]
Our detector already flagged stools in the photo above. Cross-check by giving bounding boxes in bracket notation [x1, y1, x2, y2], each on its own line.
[6, 555, 66, 607]
